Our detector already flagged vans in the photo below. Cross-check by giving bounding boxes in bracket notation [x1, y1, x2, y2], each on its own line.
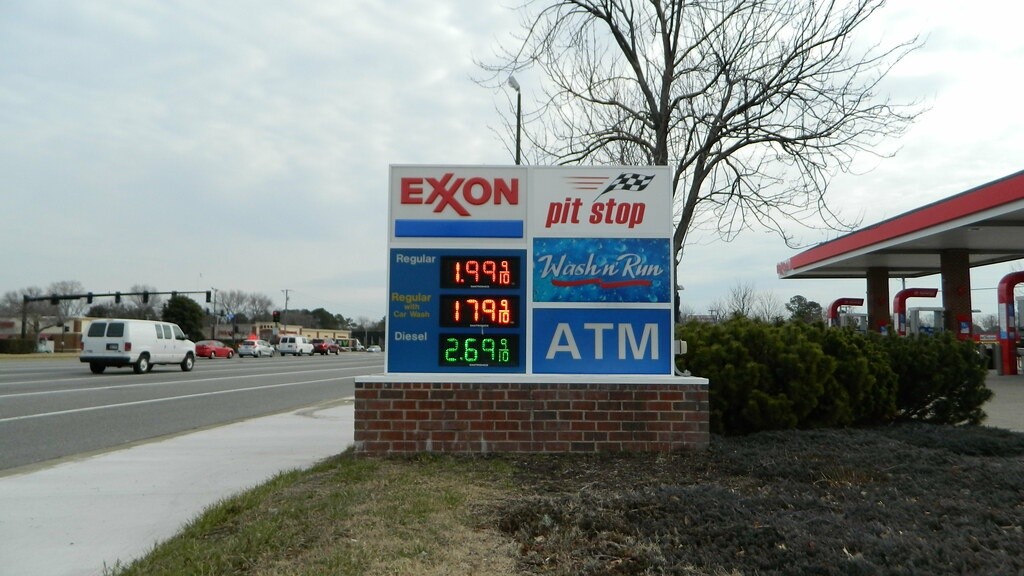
[279, 336, 314, 356]
[79, 318, 196, 374]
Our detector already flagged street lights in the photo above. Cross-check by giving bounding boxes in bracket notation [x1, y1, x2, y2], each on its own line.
[508, 75, 521, 167]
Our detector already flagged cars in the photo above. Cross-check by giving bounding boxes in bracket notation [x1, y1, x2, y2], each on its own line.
[194, 340, 235, 360]
[366, 345, 383, 353]
[311, 338, 340, 355]
[238, 340, 275, 358]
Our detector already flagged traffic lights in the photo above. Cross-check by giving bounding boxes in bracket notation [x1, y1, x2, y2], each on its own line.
[273, 311, 280, 322]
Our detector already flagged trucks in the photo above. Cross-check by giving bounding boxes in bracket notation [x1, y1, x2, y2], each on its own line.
[335, 339, 364, 351]
